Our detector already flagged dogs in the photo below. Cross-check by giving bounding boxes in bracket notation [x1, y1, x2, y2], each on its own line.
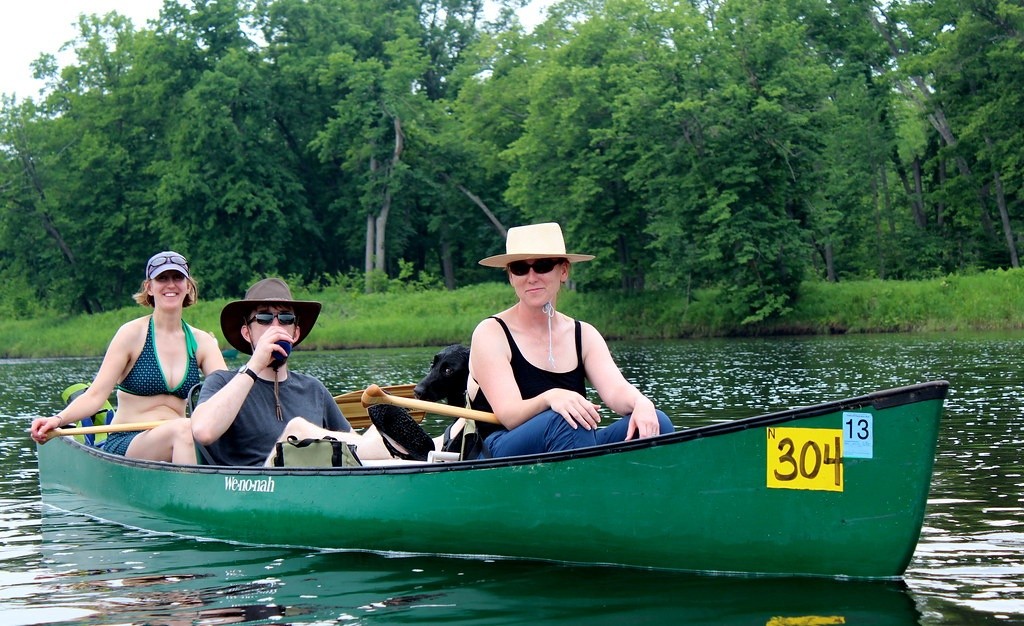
[413, 344, 471, 423]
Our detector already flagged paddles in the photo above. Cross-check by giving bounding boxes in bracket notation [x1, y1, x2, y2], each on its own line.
[36, 383, 428, 446]
[360, 385, 505, 427]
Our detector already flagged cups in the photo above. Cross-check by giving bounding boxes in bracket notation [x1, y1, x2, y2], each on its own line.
[272, 339, 292, 359]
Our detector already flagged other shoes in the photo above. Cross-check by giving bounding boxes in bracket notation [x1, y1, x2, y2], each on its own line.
[367, 389, 435, 459]
[440, 417, 466, 451]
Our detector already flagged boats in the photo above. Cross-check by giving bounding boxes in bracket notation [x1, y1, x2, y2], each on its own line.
[35, 382, 949, 579]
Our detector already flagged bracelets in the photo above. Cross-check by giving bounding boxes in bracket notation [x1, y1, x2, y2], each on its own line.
[54, 415, 62, 427]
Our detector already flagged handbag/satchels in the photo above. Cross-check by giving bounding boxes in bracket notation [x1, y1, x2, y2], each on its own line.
[271, 435, 363, 467]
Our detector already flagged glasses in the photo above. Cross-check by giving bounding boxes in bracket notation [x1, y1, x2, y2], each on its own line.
[246, 311, 297, 326]
[509, 258, 560, 276]
[148, 255, 189, 277]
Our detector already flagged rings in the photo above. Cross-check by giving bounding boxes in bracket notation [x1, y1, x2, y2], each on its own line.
[652, 434, 656, 436]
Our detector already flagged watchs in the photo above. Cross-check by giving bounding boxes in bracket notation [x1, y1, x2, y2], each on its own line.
[238, 365, 257, 383]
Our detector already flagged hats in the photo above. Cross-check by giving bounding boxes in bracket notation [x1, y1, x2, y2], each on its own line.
[146, 250, 189, 279]
[220, 278, 322, 355]
[478, 222, 596, 267]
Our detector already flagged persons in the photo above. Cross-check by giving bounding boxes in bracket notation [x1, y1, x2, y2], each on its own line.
[465, 221, 676, 460]
[190, 278, 464, 468]
[30, 250, 231, 466]
